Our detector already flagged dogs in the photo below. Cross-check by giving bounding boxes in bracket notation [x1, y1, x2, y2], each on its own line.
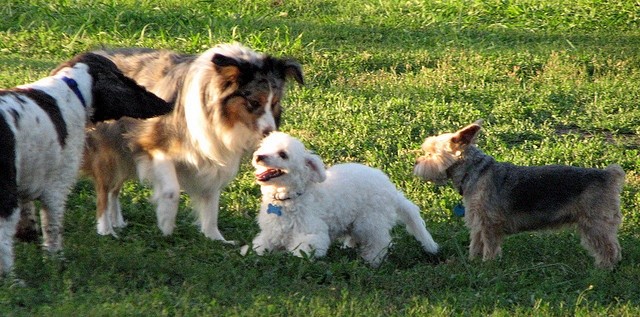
[239, 130, 440, 267]
[0, 51, 171, 280]
[49, 42, 304, 244]
[413, 120, 626, 272]
